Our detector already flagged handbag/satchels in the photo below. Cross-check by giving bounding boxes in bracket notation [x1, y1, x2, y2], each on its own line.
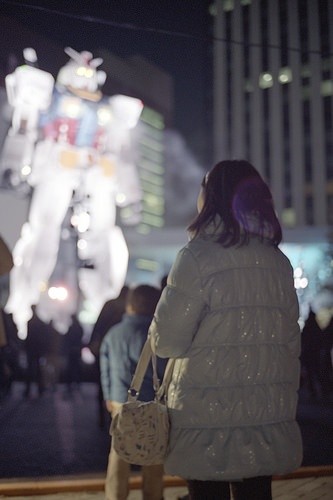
[109, 335, 170, 466]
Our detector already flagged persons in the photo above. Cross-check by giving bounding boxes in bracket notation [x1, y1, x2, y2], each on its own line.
[301, 303, 333, 406]
[0, 272, 170, 500]
[2, 47, 145, 363]
[147, 159, 302, 500]
